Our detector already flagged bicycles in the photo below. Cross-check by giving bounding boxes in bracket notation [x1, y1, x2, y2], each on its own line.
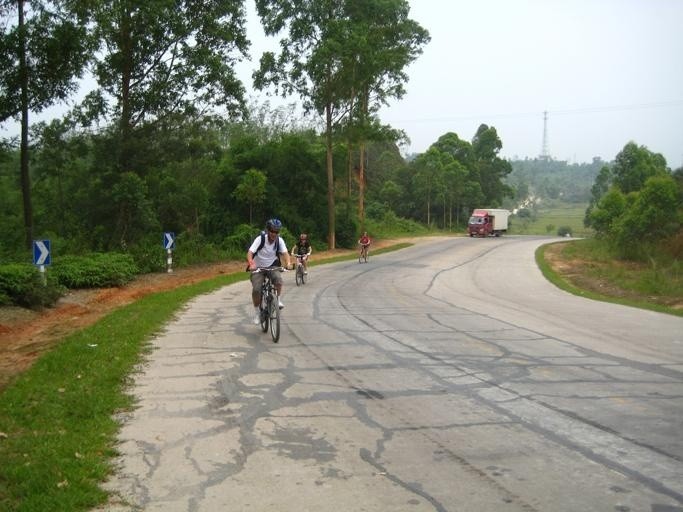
[258, 267, 285, 343]
[293, 254, 307, 286]
[359, 244, 369, 263]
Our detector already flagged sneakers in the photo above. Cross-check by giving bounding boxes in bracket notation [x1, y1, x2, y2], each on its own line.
[279, 299, 284, 310]
[254, 311, 261, 325]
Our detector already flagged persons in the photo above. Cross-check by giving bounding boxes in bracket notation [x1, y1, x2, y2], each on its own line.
[357, 230, 370, 259]
[244, 217, 293, 326]
[290, 233, 313, 275]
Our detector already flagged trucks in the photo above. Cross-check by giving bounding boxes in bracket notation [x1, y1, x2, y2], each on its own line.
[467, 209, 508, 238]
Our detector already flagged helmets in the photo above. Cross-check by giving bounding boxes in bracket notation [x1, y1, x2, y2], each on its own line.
[266, 219, 282, 231]
[300, 234, 307, 240]
